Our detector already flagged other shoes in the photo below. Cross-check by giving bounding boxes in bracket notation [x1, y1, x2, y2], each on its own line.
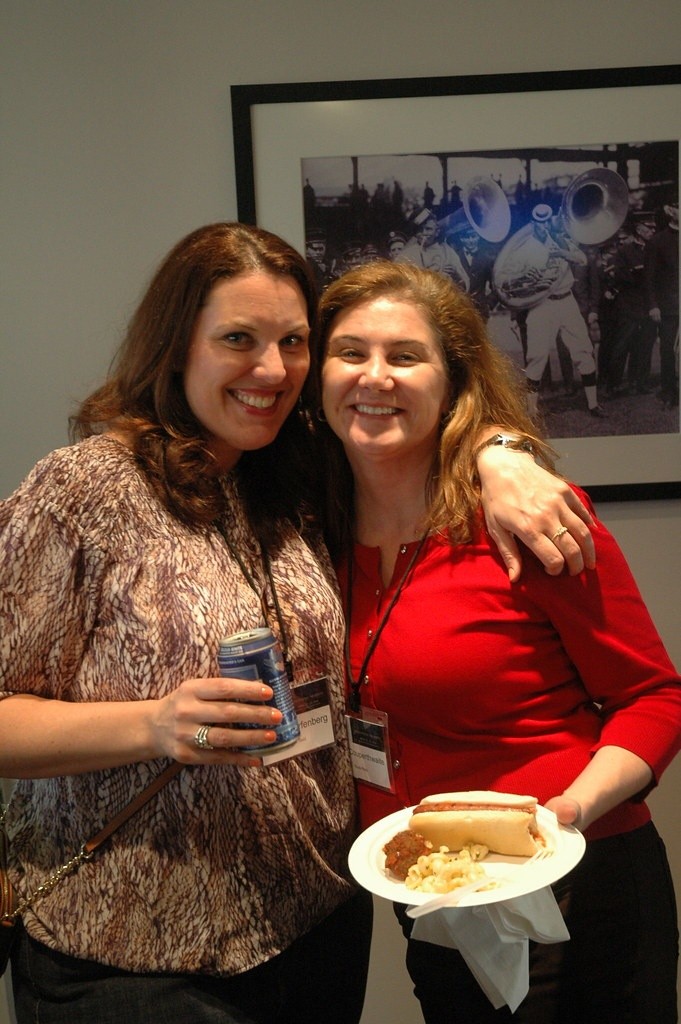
[590, 406, 608, 418]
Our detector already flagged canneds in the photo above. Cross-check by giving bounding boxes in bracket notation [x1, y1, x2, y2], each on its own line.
[216, 627, 301, 755]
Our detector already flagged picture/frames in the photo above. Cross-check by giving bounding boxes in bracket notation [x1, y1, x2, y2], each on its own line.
[231, 68, 681, 505]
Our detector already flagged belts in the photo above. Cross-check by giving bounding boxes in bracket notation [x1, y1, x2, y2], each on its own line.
[549, 292, 572, 301]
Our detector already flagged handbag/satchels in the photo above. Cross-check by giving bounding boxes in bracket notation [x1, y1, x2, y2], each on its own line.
[0, 811, 23, 975]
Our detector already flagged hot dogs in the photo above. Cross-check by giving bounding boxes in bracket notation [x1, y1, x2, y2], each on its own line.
[410, 791, 547, 857]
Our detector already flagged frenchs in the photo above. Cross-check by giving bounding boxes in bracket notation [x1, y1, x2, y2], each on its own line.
[492, 166, 630, 310]
[393, 175, 512, 277]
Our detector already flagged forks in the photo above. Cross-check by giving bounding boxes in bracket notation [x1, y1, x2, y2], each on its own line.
[406, 850, 557, 918]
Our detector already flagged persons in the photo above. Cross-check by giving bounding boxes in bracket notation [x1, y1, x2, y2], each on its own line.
[1, 222, 597, 1024]
[304, 174, 680, 418]
[304, 263, 681, 1024]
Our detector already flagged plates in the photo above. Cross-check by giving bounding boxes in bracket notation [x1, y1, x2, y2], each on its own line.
[347, 804, 586, 908]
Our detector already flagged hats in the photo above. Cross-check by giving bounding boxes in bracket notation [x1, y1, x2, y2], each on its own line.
[532, 204, 552, 221]
[338, 241, 361, 260]
[304, 225, 328, 246]
[407, 207, 435, 228]
[385, 232, 409, 249]
[633, 210, 657, 224]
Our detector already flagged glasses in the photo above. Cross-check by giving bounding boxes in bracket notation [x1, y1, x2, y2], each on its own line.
[640, 220, 657, 230]
[346, 254, 360, 260]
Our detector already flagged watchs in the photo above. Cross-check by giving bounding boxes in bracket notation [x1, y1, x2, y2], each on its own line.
[474, 433, 535, 456]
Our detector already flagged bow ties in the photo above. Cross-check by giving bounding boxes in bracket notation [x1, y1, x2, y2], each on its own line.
[465, 250, 477, 256]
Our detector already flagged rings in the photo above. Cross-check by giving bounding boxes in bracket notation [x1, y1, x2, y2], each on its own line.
[552, 527, 567, 539]
[195, 725, 213, 750]
